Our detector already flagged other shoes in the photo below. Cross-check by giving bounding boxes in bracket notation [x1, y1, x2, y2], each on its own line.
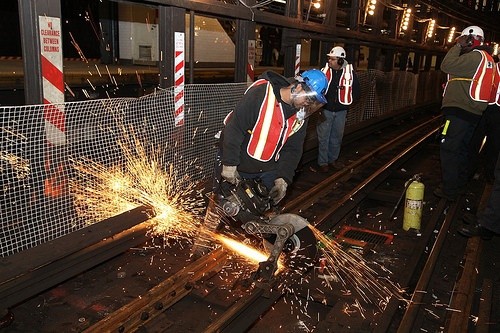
[462, 211, 477, 224]
[321, 166, 329, 172]
[434, 188, 456, 202]
[332, 163, 340, 169]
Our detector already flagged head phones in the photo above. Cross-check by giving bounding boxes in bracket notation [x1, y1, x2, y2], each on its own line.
[337, 58, 344, 66]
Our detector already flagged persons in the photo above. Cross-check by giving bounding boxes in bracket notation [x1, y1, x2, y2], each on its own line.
[433, 25, 496, 201]
[213, 69, 328, 205]
[316, 46, 362, 173]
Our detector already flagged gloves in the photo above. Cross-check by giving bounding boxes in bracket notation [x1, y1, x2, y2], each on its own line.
[269, 178, 287, 206]
[220, 164, 243, 185]
[457, 35, 470, 47]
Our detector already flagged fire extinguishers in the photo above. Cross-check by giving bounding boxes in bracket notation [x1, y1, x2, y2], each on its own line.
[402, 172, 425, 232]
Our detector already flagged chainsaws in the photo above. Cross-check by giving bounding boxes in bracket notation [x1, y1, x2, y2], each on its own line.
[218, 174, 319, 271]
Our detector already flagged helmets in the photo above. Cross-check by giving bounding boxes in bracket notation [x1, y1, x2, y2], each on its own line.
[301, 69, 328, 104]
[455, 26, 484, 45]
[327, 46, 346, 58]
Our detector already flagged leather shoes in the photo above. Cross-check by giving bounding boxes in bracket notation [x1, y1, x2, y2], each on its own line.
[457, 223, 493, 240]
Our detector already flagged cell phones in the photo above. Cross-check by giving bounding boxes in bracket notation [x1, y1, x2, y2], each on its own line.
[466, 37, 474, 46]
